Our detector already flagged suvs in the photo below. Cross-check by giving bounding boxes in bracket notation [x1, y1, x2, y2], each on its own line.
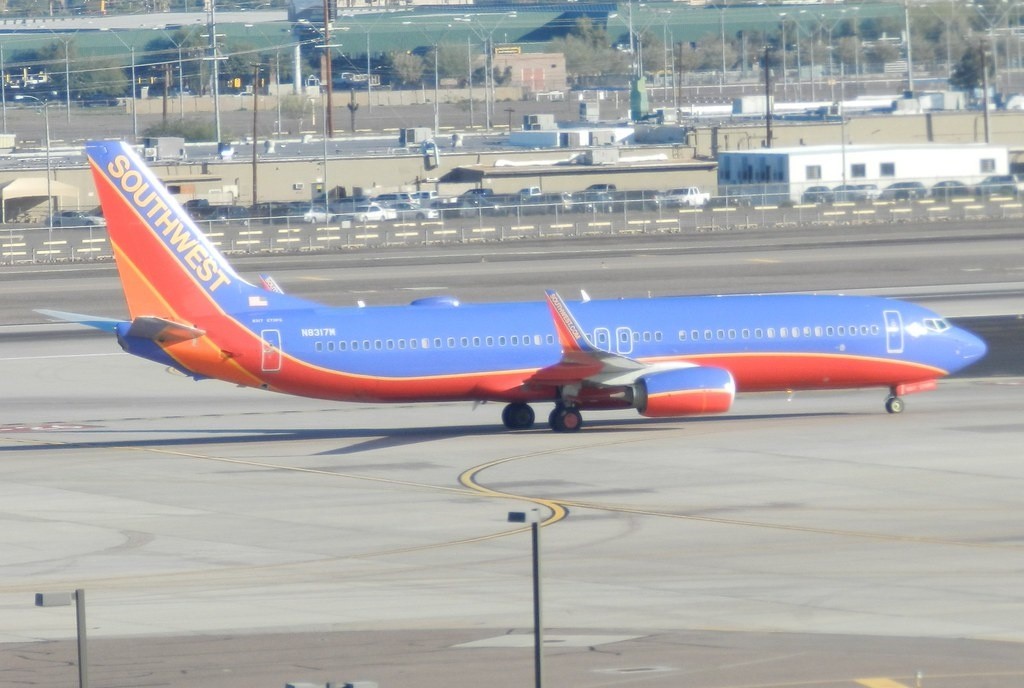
[974, 173, 1024, 198]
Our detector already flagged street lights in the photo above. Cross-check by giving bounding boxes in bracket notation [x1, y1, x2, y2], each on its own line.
[153, 22, 208, 120]
[964, 2, 1024, 94]
[99, 25, 160, 142]
[920, 3, 983, 79]
[402, 17, 463, 136]
[608, 8, 672, 78]
[342, 9, 395, 113]
[243, 21, 309, 140]
[799, 6, 861, 102]
[777, 10, 846, 103]
[455, 13, 518, 134]
[464, 11, 518, 115]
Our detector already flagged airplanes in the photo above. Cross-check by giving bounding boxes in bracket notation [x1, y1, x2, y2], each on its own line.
[28, 140, 992, 436]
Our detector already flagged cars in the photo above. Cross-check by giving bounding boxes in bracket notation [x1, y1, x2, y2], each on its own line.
[181, 182, 664, 226]
[932, 180, 969, 198]
[860, 182, 883, 201]
[801, 186, 834, 203]
[880, 182, 927, 201]
[831, 183, 868, 201]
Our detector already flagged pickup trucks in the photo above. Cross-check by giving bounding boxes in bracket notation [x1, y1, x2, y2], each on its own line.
[662, 187, 711, 209]
